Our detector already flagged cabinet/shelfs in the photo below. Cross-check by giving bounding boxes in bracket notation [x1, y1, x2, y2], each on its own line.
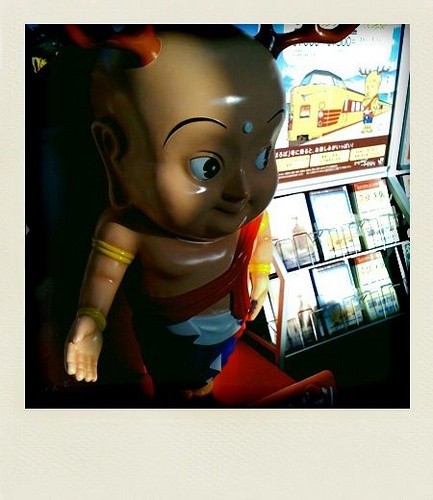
[249, 176, 410, 374]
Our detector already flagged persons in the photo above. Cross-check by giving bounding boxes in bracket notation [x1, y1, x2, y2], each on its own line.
[64, 24, 360, 409]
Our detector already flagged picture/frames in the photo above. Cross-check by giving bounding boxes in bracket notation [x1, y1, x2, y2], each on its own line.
[234, 24, 411, 189]
